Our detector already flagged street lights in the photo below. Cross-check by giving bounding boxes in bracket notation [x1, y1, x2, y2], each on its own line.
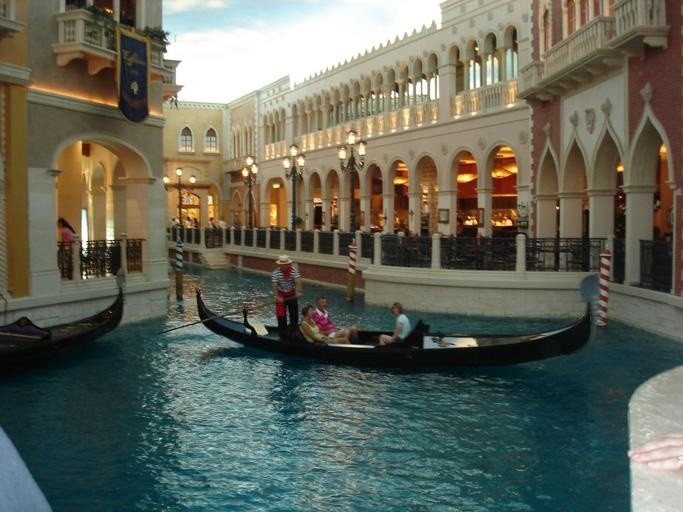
[162, 168, 196, 225]
[337, 122, 367, 232]
[282, 142, 305, 231]
[241, 155, 258, 229]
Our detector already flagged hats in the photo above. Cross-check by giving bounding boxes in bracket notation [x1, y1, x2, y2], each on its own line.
[276, 255, 293, 265]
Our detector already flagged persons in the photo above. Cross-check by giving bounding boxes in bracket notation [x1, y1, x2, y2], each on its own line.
[171, 211, 242, 248]
[311, 295, 360, 346]
[56, 217, 76, 281]
[299, 304, 353, 345]
[377, 302, 411, 346]
[270, 254, 302, 330]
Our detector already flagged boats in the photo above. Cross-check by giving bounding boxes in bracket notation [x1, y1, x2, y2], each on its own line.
[196, 288, 594, 369]
[0, 287, 124, 369]
